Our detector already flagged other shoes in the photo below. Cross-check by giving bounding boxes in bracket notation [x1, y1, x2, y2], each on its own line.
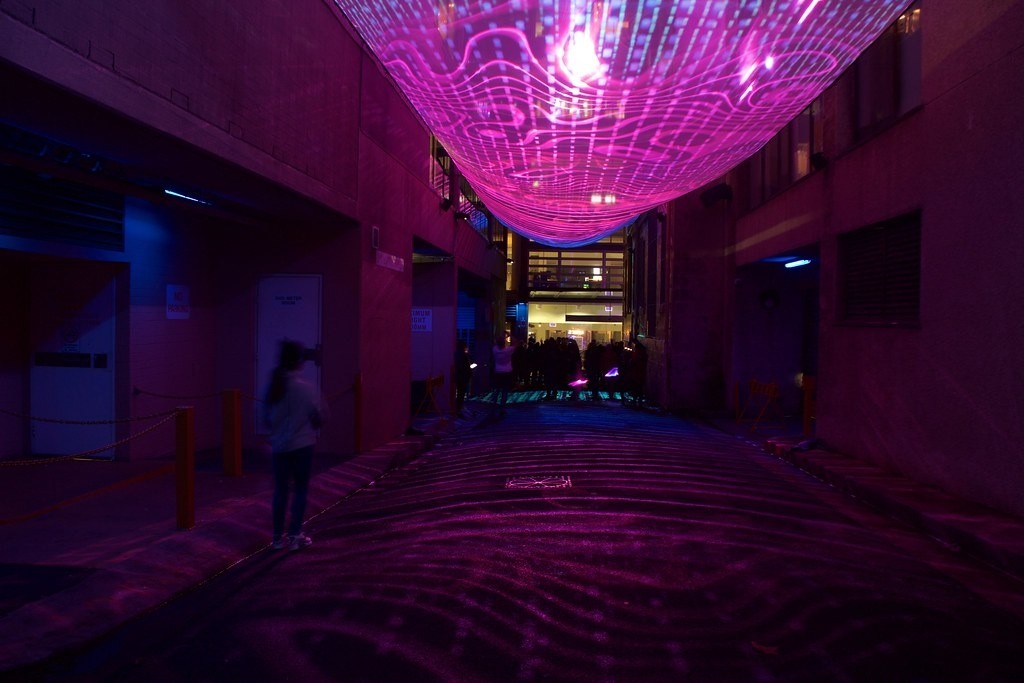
[499, 408, 506, 414]
[457, 410, 463, 417]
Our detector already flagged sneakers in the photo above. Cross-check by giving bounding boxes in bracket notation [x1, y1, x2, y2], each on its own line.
[287, 532, 312, 551]
[273, 533, 290, 549]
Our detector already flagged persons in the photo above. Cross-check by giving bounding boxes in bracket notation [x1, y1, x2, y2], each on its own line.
[264, 340, 324, 553]
[515, 336, 580, 401]
[491, 336, 518, 415]
[584, 337, 647, 398]
[455, 341, 470, 418]
